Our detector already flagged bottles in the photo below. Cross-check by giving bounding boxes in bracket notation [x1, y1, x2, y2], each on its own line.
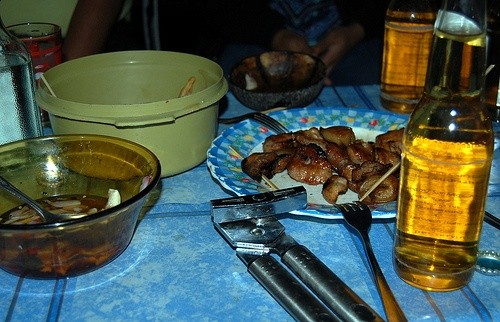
[0, 15, 44, 147]
[393, 0, 494, 293]
[380, 0, 441, 113]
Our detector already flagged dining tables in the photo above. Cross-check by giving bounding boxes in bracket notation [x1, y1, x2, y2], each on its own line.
[0, 85, 500, 322]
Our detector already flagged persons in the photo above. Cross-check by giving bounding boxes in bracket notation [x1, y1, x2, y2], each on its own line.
[0, 0, 121, 62]
[257, 0, 391, 87]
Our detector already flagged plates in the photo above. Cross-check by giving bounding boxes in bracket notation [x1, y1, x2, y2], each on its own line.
[207, 106, 410, 219]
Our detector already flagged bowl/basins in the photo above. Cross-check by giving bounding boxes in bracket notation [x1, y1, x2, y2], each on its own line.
[228, 50, 326, 111]
[0, 133, 162, 280]
[35, 49, 229, 180]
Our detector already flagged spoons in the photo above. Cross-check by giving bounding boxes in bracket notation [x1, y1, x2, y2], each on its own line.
[0, 175, 107, 248]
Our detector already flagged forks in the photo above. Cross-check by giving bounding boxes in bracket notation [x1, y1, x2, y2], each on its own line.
[218, 113, 289, 135]
[334, 201, 408, 322]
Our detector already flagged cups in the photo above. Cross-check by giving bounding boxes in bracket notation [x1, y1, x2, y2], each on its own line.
[6, 22, 62, 125]
